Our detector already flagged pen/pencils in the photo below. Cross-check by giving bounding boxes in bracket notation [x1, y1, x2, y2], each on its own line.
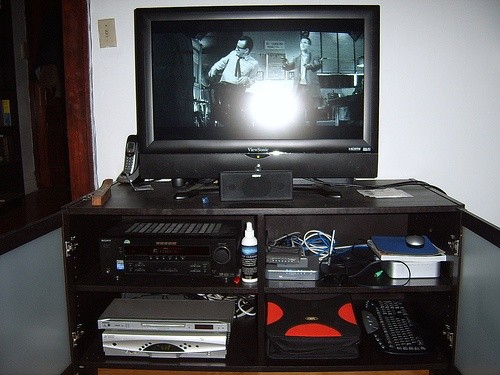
[233, 269, 241, 284]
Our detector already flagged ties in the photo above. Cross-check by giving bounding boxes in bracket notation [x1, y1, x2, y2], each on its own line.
[235, 57, 241, 78]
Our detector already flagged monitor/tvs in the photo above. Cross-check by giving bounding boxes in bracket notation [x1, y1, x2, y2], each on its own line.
[135, 4, 380, 197]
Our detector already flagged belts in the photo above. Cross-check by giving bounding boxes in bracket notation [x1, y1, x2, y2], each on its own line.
[224, 82, 234, 87]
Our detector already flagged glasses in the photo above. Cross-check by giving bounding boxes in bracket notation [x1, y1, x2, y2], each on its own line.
[234, 45, 246, 50]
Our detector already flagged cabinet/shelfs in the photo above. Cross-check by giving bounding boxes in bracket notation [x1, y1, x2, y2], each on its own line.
[60, 180, 465, 375]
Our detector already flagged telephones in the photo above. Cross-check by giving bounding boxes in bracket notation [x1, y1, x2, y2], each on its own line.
[116, 134, 141, 184]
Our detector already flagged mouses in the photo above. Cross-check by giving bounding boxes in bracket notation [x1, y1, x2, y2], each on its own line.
[406, 235, 425, 248]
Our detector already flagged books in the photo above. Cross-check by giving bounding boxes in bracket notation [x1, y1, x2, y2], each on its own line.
[367, 237, 447, 262]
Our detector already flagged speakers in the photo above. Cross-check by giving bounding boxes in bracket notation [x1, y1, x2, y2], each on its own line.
[220, 170, 293, 201]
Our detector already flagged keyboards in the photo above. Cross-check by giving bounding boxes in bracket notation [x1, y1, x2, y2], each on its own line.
[362, 300, 427, 356]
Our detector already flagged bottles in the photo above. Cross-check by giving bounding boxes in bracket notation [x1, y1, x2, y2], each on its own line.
[241, 222, 258, 283]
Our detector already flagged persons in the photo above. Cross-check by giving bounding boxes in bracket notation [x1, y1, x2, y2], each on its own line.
[281, 38, 322, 127]
[208, 36, 259, 117]
